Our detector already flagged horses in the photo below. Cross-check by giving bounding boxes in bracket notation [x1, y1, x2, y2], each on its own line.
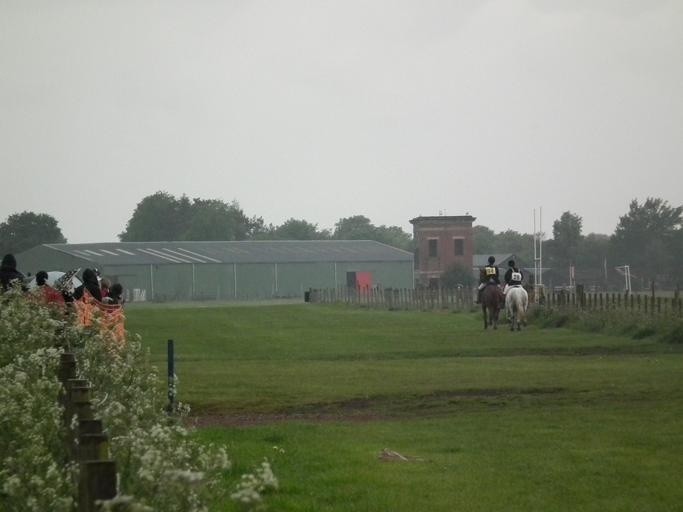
[502, 281, 529, 331]
[477, 267, 506, 330]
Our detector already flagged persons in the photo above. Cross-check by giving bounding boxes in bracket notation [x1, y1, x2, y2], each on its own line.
[501, 259, 524, 302]
[470, 255, 503, 305]
[0, 251, 127, 347]
